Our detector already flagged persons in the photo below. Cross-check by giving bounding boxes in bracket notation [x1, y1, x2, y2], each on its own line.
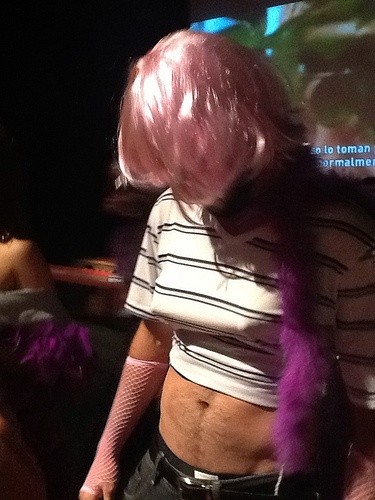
[77, 29, 375, 499]
[1, 171, 64, 313]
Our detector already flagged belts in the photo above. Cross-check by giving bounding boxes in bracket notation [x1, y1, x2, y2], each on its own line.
[149, 430, 317, 500]
